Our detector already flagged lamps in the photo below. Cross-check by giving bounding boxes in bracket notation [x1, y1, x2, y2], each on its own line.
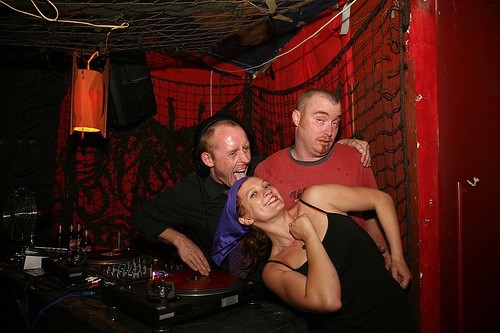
[68, 41, 110, 140]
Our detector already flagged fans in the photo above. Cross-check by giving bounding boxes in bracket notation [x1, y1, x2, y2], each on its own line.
[2, 183, 48, 242]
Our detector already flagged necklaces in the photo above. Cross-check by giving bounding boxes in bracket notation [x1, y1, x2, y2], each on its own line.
[271, 211, 306, 248]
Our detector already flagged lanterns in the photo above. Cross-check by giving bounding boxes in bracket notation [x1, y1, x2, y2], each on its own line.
[71, 50, 110, 141]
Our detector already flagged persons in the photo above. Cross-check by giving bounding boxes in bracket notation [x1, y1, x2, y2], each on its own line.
[132, 114, 370, 299]
[253, 88, 392, 269]
[210, 175, 421, 332]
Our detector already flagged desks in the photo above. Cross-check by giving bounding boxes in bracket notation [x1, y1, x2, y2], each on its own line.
[27, 284, 307, 333]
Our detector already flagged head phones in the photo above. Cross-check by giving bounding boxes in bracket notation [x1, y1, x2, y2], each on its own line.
[194, 116, 234, 179]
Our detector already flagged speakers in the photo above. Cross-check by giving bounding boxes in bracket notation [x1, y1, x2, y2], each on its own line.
[90, 49, 157, 132]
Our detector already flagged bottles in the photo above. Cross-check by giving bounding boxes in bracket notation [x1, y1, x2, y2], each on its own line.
[82, 227, 92, 252]
[76, 222, 83, 252]
[57, 224, 62, 248]
[68, 224, 77, 252]
